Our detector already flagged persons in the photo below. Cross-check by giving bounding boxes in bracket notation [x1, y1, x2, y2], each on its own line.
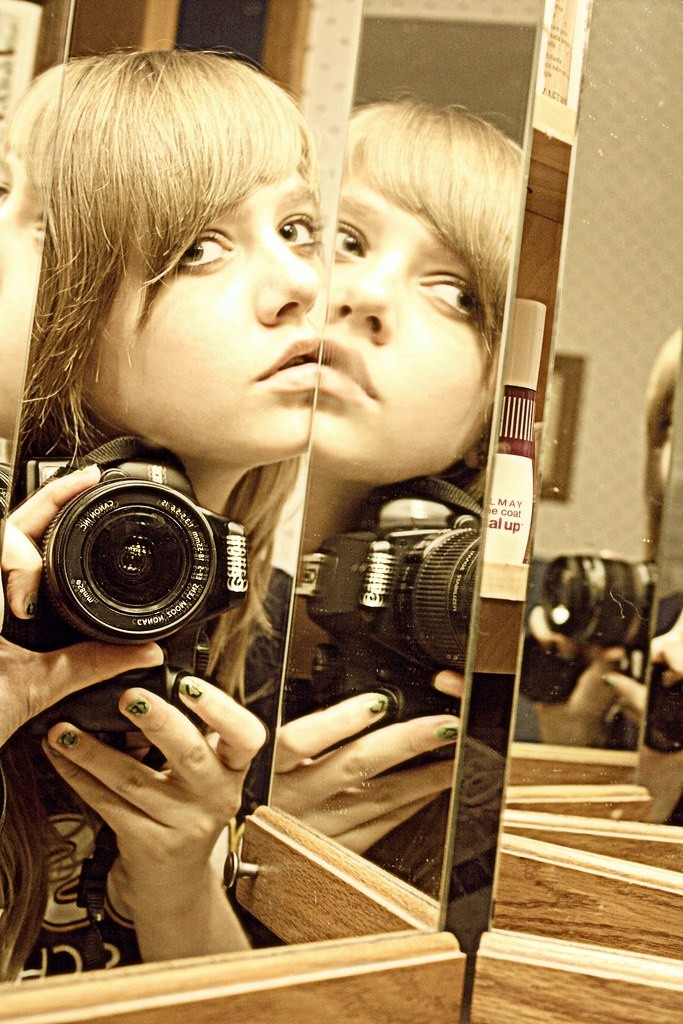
[0, 45, 682, 981]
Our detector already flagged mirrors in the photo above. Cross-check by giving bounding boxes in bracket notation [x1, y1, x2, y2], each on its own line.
[1, 0, 683, 980]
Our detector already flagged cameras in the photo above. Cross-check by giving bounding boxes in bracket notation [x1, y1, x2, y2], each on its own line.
[8, 457, 249, 688]
[295, 507, 658, 781]
